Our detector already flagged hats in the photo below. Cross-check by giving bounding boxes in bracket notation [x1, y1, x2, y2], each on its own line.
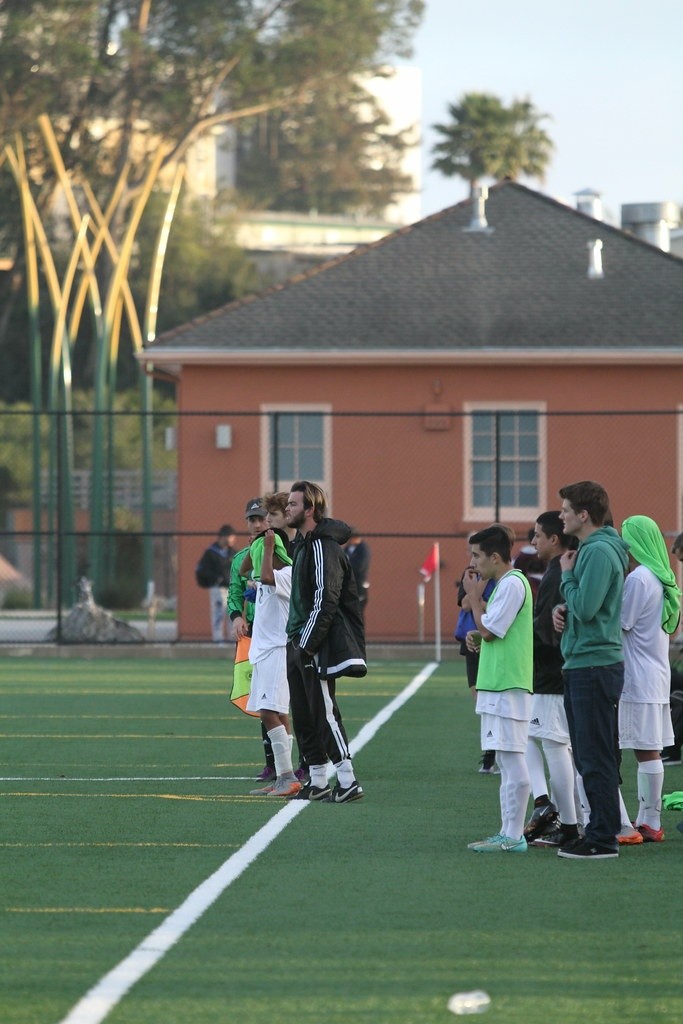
[244, 501, 268, 518]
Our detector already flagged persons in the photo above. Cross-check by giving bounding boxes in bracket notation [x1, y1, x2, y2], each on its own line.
[348, 528, 369, 618]
[462, 527, 533, 853]
[285, 480, 369, 803]
[455, 482, 683, 858]
[240, 527, 302, 796]
[227, 498, 270, 776]
[202, 525, 238, 645]
[251, 491, 310, 783]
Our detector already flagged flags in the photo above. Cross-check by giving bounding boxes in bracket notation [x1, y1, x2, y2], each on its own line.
[419, 546, 437, 582]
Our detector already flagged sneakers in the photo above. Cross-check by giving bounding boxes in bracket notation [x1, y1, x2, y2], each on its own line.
[287, 778, 331, 800]
[473, 835, 529, 852]
[558, 838, 619, 858]
[536, 827, 581, 844]
[659, 750, 671, 758]
[662, 756, 682, 765]
[539, 814, 563, 838]
[467, 833, 501, 848]
[294, 765, 311, 781]
[322, 778, 364, 803]
[523, 800, 560, 842]
[250, 779, 278, 795]
[631, 821, 666, 843]
[256, 766, 277, 783]
[616, 824, 644, 844]
[268, 777, 301, 798]
[478, 750, 496, 772]
